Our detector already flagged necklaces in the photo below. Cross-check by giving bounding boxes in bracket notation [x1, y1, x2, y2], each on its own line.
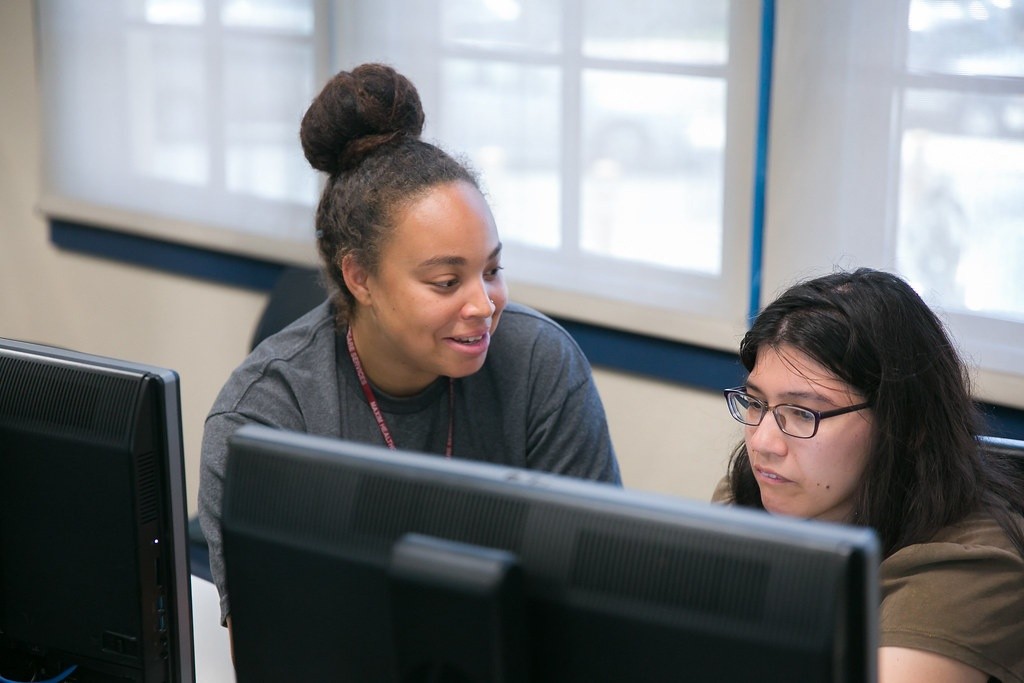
[346, 323, 456, 457]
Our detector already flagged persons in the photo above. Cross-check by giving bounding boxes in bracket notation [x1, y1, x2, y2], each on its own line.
[709, 268, 1024, 683]
[187, 62, 625, 664]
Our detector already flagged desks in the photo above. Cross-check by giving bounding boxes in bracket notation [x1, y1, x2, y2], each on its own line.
[190, 575, 237, 683]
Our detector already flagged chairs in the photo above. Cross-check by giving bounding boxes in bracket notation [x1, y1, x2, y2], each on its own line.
[183, 266, 336, 585]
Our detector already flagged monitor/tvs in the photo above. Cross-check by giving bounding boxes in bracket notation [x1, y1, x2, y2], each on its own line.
[220, 421, 881, 683]
[0, 337, 196, 683]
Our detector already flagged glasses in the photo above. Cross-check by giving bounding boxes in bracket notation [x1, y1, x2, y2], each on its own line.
[723, 386, 876, 439]
